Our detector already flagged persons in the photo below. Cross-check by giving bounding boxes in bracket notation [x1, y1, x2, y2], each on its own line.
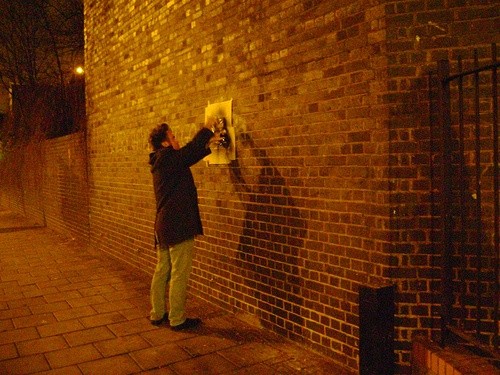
[149, 115, 220, 330]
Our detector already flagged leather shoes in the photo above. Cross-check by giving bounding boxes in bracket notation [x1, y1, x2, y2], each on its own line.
[150, 312, 168, 325]
[171, 318, 201, 331]
